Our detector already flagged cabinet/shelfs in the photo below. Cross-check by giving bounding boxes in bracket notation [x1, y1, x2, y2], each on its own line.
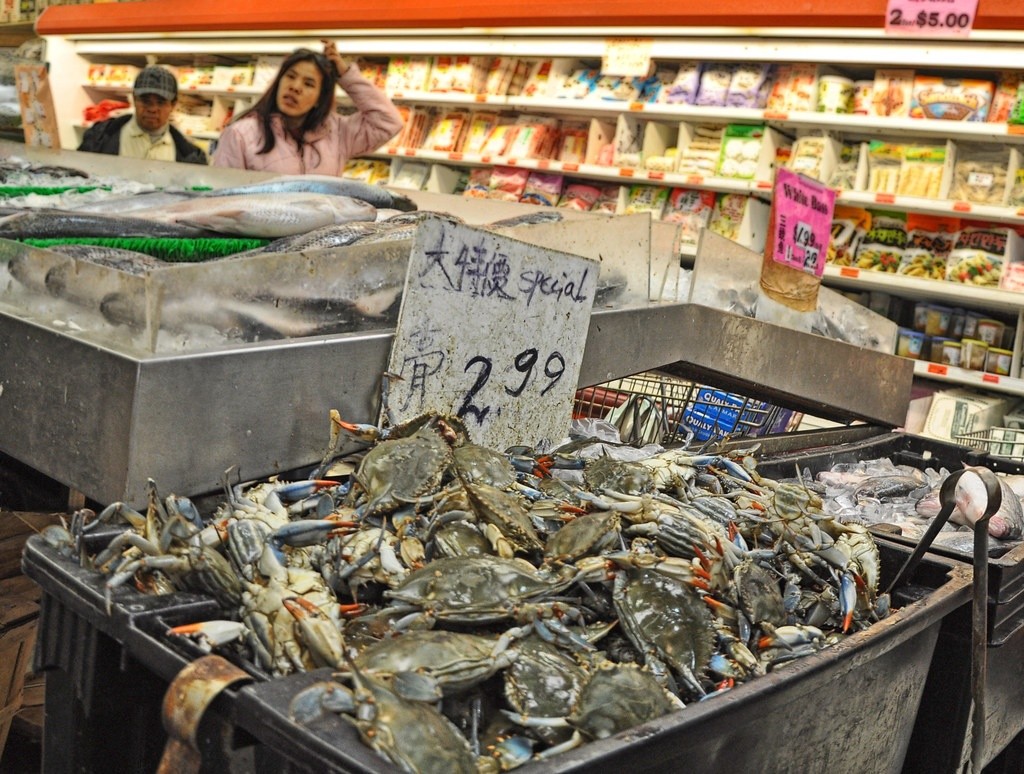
[33, 0, 1023, 473]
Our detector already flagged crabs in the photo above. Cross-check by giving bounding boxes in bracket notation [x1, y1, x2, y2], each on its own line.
[51, 410, 893, 774]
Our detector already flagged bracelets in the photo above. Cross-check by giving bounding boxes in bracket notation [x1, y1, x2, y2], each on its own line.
[339, 66, 351, 77]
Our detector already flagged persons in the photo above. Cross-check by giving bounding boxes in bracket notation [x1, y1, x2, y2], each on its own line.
[209, 38, 404, 177]
[76, 67, 208, 166]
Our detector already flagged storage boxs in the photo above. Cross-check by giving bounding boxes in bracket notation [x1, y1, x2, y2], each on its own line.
[681, 388, 802, 441]
[874, 69, 995, 122]
[22, 428, 1022, 774]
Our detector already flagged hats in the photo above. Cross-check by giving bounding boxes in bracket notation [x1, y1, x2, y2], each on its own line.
[132, 66, 177, 102]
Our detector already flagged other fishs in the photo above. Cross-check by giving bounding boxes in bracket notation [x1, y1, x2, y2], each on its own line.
[0, 155, 566, 342]
[782, 456, 1024, 560]
